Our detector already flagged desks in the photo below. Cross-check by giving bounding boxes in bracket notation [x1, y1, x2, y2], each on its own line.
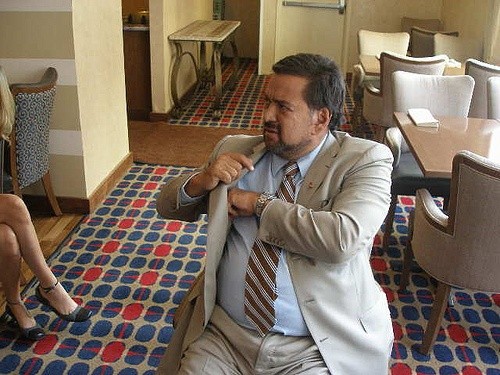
[359, 54, 465, 75]
[169, 19, 241, 119]
[393, 112, 500, 177]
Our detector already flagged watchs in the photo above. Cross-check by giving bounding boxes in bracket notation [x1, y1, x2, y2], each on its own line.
[256, 191, 277, 218]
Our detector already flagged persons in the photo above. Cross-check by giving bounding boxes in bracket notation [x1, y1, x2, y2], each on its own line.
[158, 52, 395, 375]
[0, 65, 92, 341]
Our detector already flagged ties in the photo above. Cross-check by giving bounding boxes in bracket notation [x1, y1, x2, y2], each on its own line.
[244, 158, 300, 338]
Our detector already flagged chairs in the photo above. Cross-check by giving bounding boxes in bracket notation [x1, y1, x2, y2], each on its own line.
[402, 17, 441, 43]
[399, 150, 500, 355]
[435, 34, 486, 64]
[363, 53, 448, 141]
[411, 26, 459, 57]
[464, 58, 500, 119]
[354, 28, 411, 89]
[381, 71, 476, 249]
[0, 66, 64, 216]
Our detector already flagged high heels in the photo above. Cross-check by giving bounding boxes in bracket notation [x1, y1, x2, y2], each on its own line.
[35, 279, 92, 321]
[5, 302, 45, 339]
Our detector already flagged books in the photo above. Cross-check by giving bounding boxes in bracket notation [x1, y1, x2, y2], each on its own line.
[408, 108, 439, 128]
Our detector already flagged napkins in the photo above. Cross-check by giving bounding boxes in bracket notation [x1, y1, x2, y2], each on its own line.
[408, 108, 439, 127]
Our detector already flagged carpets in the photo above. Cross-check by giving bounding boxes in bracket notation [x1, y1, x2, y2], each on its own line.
[128, 122, 262, 167]
